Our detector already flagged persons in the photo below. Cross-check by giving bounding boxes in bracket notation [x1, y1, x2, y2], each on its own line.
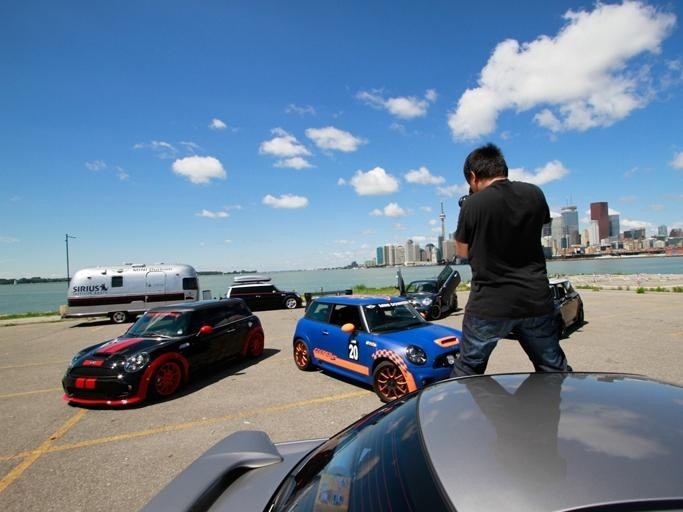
[455, 371, 576, 511]
[450, 142, 574, 378]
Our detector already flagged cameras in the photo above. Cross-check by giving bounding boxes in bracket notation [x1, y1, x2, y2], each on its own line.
[458, 187, 473, 207]
